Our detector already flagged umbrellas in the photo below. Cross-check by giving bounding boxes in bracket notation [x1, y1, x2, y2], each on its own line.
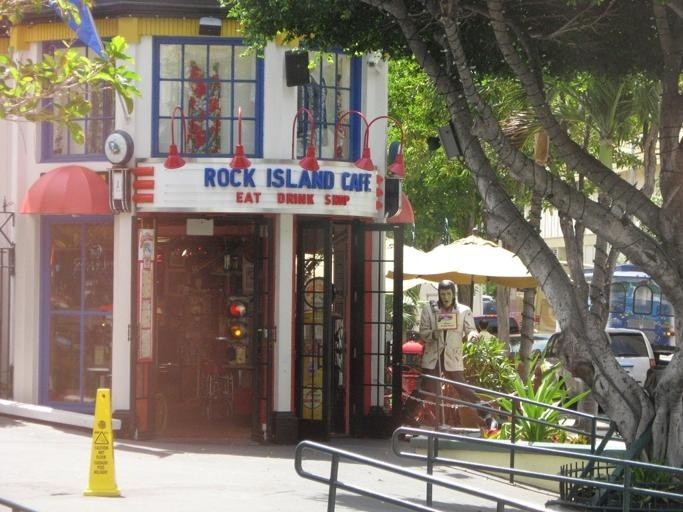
[381, 234, 542, 322]
[379, 235, 432, 303]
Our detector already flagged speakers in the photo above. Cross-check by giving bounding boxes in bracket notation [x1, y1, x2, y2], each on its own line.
[285, 49, 310, 87]
[198, 17, 223, 36]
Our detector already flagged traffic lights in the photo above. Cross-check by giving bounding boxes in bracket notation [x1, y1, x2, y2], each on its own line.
[224, 295, 249, 348]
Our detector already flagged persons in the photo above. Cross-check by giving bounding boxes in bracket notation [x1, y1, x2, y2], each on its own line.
[397, 278, 505, 435]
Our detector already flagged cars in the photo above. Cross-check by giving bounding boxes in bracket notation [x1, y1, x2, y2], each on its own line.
[401, 294, 677, 431]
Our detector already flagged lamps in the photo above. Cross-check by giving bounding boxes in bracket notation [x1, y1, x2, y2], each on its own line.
[292, 108, 320, 171]
[364, 115, 405, 176]
[164, 105, 186, 169]
[334, 111, 374, 170]
[229, 106, 251, 170]
